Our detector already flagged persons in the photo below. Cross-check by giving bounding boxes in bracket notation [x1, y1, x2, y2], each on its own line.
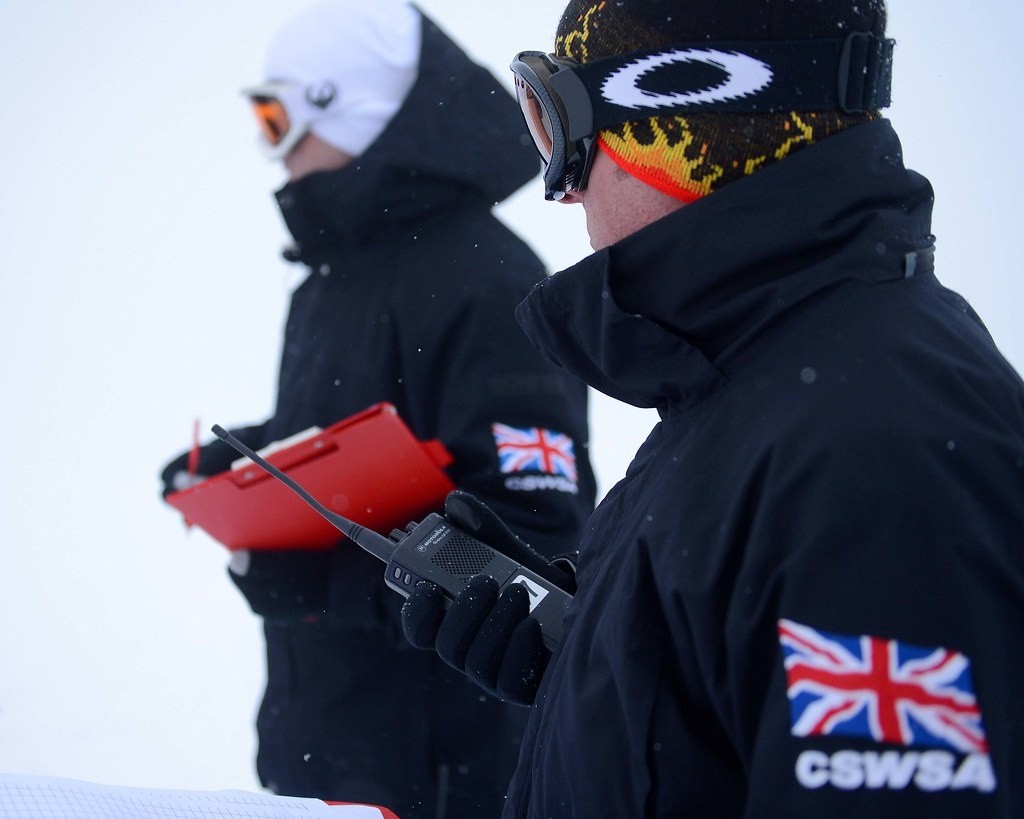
[158, 0, 597, 819]
[402, 0, 1024, 819]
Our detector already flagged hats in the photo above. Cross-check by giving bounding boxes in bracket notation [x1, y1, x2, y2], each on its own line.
[245, 0, 419, 157]
[554, 0, 888, 203]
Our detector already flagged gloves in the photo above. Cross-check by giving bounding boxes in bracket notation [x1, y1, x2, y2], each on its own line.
[402, 490, 578, 707]
[230, 546, 327, 618]
[163, 426, 262, 495]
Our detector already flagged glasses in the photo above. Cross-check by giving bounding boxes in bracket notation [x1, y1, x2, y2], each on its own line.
[510, 49, 598, 200]
[247, 85, 308, 158]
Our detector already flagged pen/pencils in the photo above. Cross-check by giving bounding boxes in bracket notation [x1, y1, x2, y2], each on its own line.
[184, 420, 199, 530]
